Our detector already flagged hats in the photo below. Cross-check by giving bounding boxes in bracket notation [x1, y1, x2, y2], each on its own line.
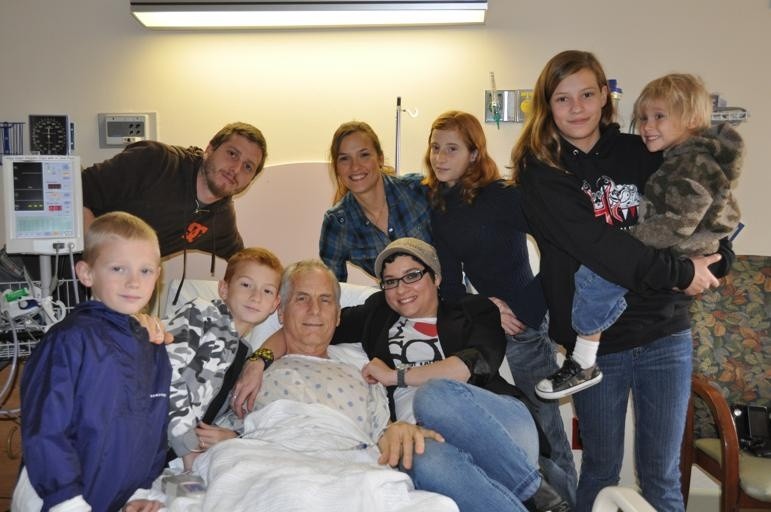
[375, 238, 441, 279]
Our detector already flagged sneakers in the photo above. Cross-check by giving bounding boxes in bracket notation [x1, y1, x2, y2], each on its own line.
[534, 356, 603, 400]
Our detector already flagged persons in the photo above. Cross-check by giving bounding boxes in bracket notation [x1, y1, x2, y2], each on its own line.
[17, 212, 171, 512]
[533, 71, 747, 401]
[419, 109, 581, 512]
[119, 257, 447, 512]
[509, 47, 738, 512]
[225, 236, 581, 512]
[158, 242, 283, 477]
[317, 117, 465, 295]
[1, 121, 269, 349]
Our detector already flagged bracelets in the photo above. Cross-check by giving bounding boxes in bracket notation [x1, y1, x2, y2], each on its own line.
[246, 349, 275, 371]
[397, 364, 412, 389]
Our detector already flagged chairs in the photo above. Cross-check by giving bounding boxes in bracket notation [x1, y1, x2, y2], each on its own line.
[680, 255, 771, 512]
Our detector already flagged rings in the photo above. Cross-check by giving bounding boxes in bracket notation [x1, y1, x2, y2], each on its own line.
[232, 393, 238, 402]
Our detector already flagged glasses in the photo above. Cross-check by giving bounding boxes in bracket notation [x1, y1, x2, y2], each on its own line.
[380, 268, 427, 290]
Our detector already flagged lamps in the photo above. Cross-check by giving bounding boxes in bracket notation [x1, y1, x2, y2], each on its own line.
[129, 2, 490, 29]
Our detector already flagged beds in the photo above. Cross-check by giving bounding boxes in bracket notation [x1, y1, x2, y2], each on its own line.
[164, 161, 659, 512]
